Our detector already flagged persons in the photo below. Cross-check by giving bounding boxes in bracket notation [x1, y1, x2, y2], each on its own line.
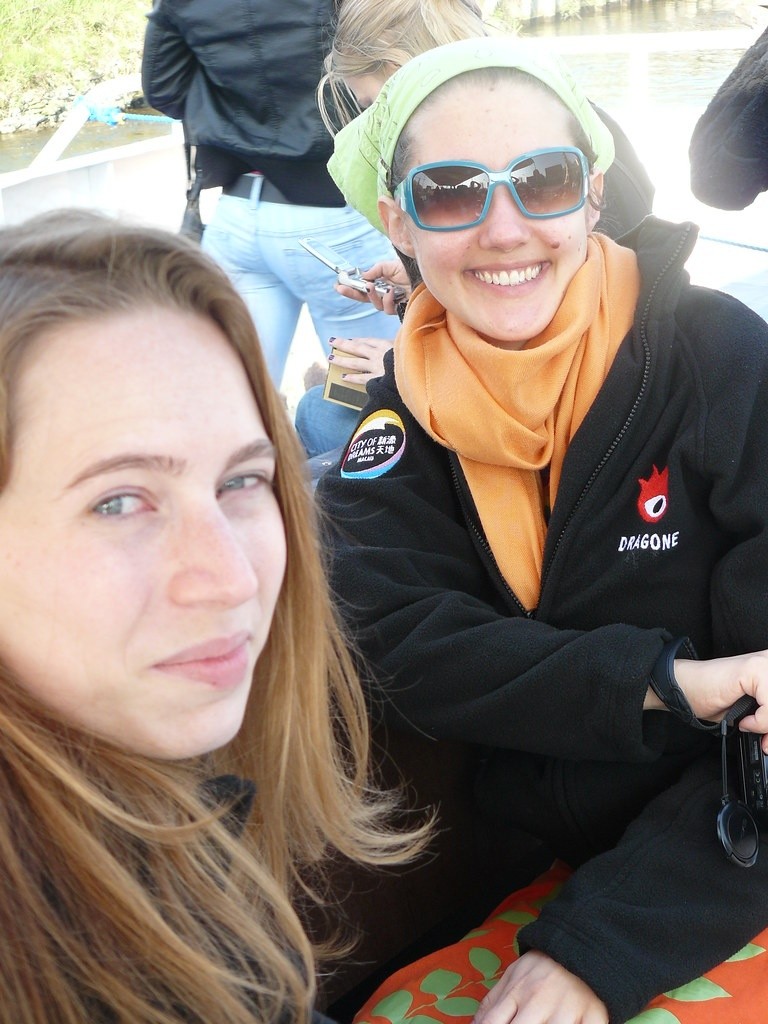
[0, 208, 439, 1024]
[0, 0, 768, 1024]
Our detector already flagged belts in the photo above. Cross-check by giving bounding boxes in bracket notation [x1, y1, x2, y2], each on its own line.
[221, 174, 298, 205]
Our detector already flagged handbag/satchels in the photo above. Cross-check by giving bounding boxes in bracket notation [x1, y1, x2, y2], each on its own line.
[174, 120, 209, 242]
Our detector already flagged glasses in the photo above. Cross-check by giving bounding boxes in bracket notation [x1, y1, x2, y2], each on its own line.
[394, 146, 591, 232]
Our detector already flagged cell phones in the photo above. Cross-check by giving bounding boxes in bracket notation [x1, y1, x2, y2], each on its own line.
[299, 237, 405, 299]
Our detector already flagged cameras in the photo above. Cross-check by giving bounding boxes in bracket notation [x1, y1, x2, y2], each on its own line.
[735, 700, 768, 822]
